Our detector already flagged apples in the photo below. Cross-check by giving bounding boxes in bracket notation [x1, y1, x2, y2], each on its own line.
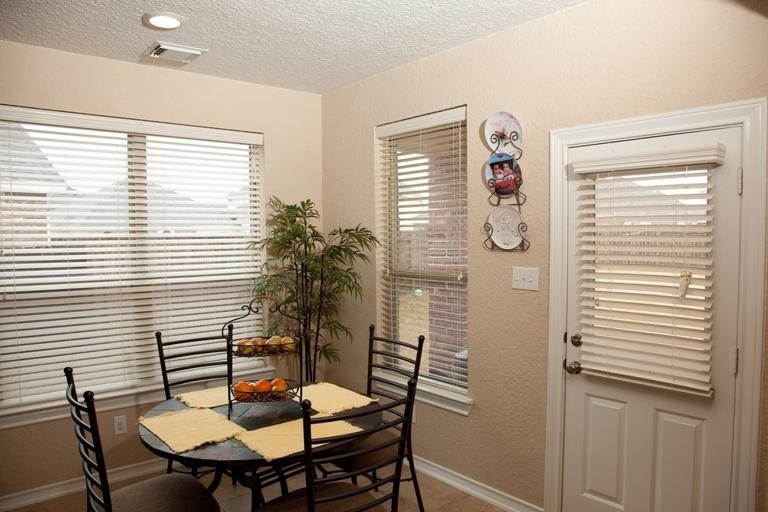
[253, 338, 264, 352]
[239, 340, 253, 354]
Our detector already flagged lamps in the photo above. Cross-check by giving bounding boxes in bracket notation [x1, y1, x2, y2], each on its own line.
[140, 9, 185, 32]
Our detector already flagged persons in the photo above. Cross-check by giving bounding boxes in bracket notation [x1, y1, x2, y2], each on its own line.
[503, 163, 516, 181]
[492, 163, 504, 181]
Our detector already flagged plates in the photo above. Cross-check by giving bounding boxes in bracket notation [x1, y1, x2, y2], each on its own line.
[488, 205, 525, 250]
[484, 112, 523, 154]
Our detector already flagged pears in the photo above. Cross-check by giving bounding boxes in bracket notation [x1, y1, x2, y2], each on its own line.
[265, 335, 281, 353]
[281, 336, 295, 351]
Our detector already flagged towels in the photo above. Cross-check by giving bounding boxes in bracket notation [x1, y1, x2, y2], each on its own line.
[138, 408, 245, 454]
[175, 384, 243, 410]
[235, 413, 364, 462]
[286, 382, 378, 412]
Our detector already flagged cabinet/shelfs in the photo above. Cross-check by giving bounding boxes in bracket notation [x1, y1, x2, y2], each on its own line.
[222, 295, 306, 406]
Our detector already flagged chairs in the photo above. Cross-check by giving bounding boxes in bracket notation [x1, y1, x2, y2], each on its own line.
[324, 323, 425, 511]
[63, 366, 221, 512]
[255, 378, 417, 512]
[155, 322, 238, 488]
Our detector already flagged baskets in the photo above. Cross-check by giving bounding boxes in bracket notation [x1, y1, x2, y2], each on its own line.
[231, 377, 298, 402]
[230, 336, 299, 358]
